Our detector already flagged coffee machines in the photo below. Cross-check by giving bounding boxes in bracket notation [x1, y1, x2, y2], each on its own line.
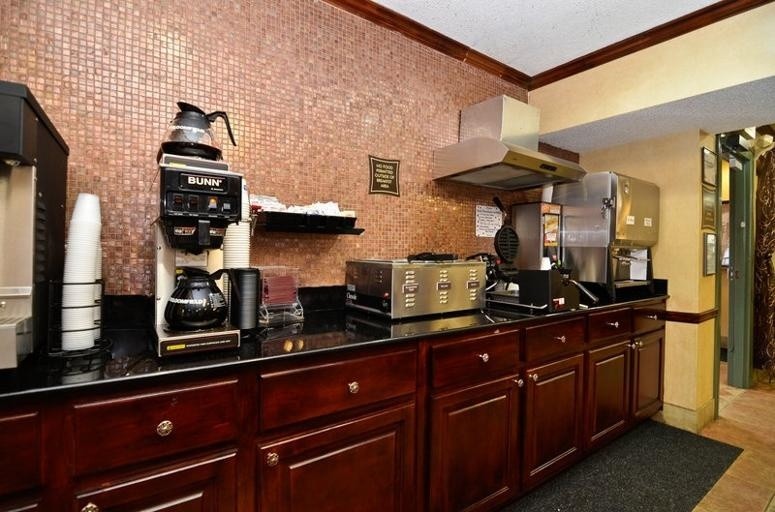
[146, 154, 245, 363]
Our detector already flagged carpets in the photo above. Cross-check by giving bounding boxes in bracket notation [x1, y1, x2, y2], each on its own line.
[500, 417, 745, 511]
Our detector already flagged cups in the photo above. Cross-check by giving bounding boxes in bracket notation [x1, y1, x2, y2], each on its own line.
[61, 193, 102, 351]
[223, 178, 250, 317]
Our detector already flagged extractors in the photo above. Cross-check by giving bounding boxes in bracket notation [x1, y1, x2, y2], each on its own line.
[432, 94, 588, 191]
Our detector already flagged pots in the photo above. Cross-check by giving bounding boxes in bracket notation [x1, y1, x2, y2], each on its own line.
[491, 195, 521, 264]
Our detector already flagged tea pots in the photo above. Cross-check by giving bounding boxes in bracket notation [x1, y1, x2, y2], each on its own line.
[163, 266, 242, 330]
[161, 99, 237, 163]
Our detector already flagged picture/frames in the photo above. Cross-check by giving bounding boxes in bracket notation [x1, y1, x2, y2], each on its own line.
[702, 147, 720, 277]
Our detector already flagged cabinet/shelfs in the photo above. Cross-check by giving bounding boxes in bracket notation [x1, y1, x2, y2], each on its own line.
[585, 295, 670, 457]
[421, 302, 585, 512]
[64, 349, 258, 511]
[258, 331, 421, 512]
